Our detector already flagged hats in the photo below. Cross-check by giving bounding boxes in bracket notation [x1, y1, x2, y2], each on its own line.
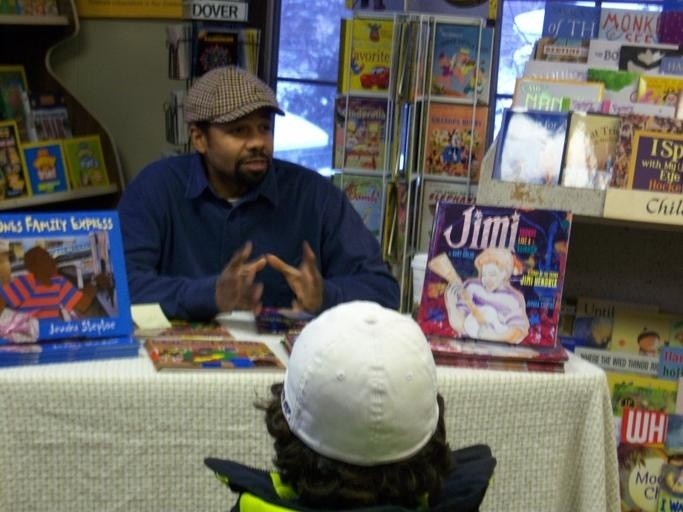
[180, 63, 287, 125]
[280, 295, 444, 469]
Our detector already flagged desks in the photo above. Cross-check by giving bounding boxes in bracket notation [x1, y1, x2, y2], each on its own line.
[0, 300, 621, 512]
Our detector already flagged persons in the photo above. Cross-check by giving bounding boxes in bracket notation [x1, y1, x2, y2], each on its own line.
[205, 299, 497, 512]
[116, 64, 400, 323]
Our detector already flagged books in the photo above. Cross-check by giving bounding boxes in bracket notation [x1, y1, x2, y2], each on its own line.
[333, 1, 682, 374]
[144, 337, 287, 373]
[254, 307, 317, 335]
[279, 327, 306, 357]
[1, 62, 141, 370]
[163, 22, 261, 161]
[557, 296, 682, 512]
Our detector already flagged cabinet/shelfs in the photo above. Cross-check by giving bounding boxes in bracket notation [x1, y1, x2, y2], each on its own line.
[330, 12, 484, 321]
[162, 18, 264, 160]
[475, 36, 683, 512]
[0, 0, 130, 304]
